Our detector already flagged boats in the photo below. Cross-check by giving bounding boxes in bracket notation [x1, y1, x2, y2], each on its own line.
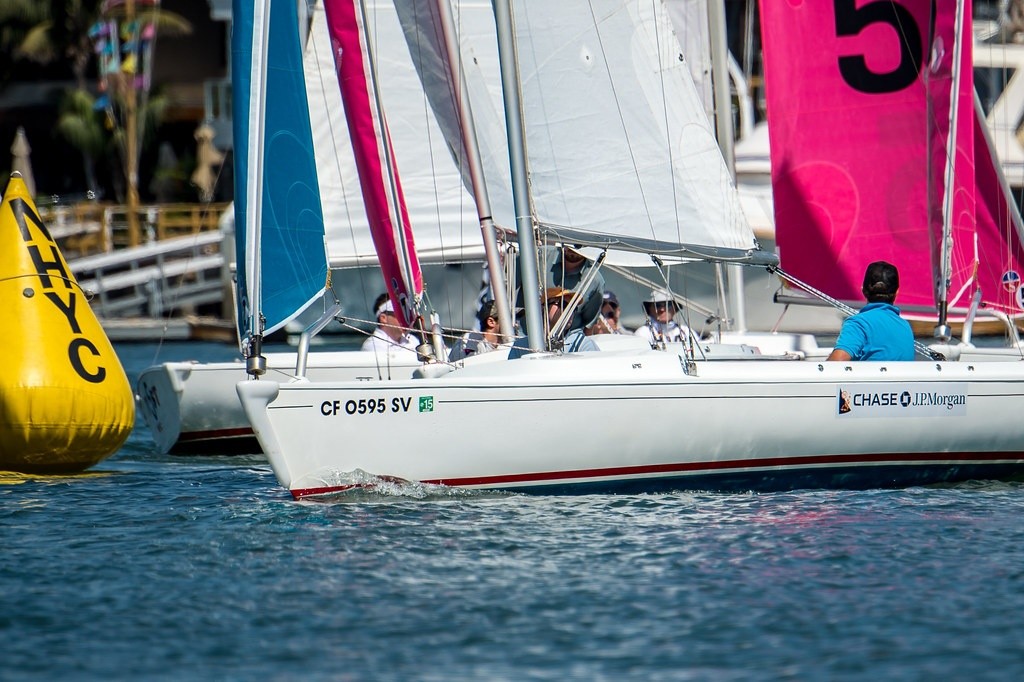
[218, 36, 1024, 357]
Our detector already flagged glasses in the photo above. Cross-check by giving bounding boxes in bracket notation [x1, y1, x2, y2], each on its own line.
[542, 299, 578, 312]
[653, 303, 674, 309]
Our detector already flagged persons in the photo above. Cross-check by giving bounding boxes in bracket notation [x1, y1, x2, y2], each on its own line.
[635, 290, 703, 342]
[552, 247, 605, 330]
[361, 294, 419, 350]
[826, 261, 915, 361]
[508, 287, 601, 360]
[583, 290, 632, 335]
[448, 300, 525, 362]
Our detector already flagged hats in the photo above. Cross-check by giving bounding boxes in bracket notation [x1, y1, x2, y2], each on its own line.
[864, 261, 898, 293]
[376, 299, 395, 318]
[541, 288, 584, 305]
[643, 290, 682, 316]
[479, 300, 525, 322]
[602, 290, 617, 305]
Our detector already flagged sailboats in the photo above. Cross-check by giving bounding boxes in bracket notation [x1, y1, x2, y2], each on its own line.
[135, 0, 523, 449]
[235, 1, 1023, 494]
